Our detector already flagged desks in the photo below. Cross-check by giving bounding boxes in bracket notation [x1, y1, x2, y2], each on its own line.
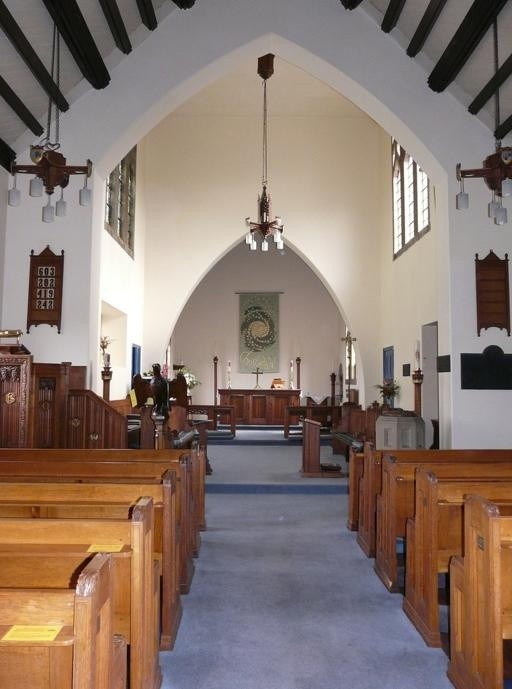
[218, 389, 301, 424]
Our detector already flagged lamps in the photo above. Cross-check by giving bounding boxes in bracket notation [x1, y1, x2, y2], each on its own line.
[456, 14, 511, 224]
[8, 25, 92, 221]
[246, 77, 284, 251]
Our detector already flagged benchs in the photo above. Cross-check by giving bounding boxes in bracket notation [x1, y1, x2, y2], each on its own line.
[403, 468, 511, 648]
[0, 448, 204, 689]
[357, 444, 510, 557]
[446, 494, 511, 687]
[374, 450, 511, 593]
[349, 444, 360, 531]
